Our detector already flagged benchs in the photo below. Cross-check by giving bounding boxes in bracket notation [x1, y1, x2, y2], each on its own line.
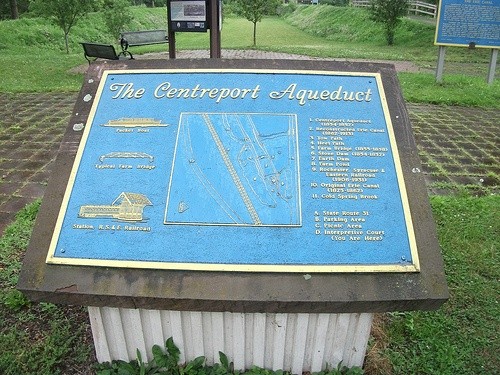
[119, 30, 169, 57]
[78, 42, 133, 65]
